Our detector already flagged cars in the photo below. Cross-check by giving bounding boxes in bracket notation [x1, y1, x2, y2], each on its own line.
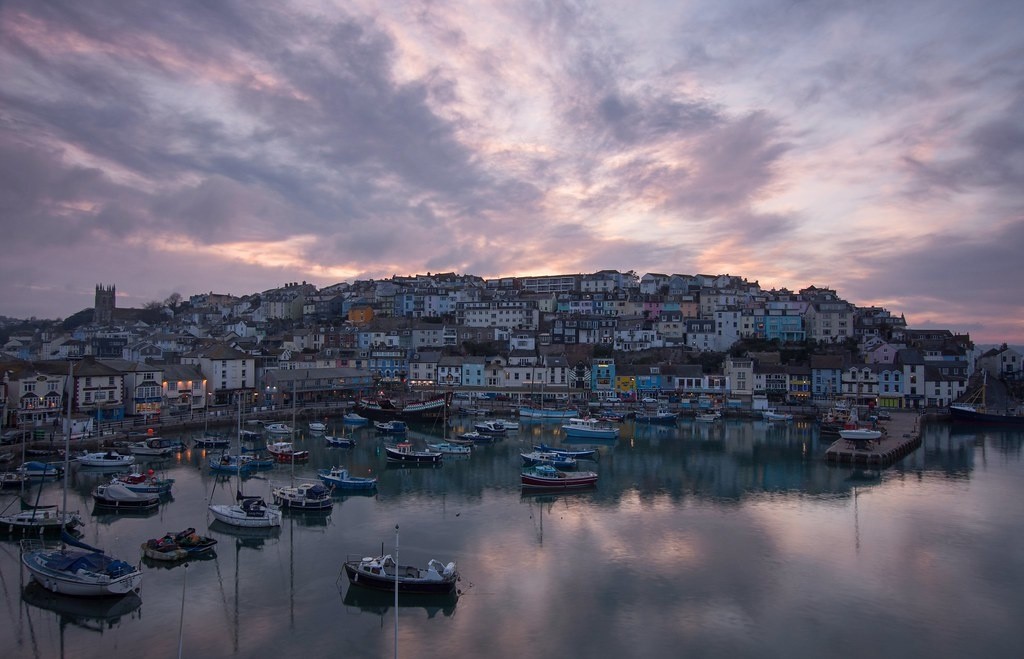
[605, 396, 622, 403]
[455, 393, 469, 399]
[622, 397, 636, 403]
[562, 396, 578, 403]
[495, 394, 512, 401]
[641, 396, 658, 403]
[475, 393, 491, 401]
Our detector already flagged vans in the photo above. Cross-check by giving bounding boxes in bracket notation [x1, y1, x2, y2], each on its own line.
[0, 429, 32, 445]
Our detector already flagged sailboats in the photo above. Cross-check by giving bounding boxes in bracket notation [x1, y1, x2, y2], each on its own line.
[271, 377, 336, 511]
[20, 360, 144, 596]
[207, 391, 282, 528]
[424, 387, 472, 454]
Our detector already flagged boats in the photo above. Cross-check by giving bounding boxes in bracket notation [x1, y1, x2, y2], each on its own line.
[761, 411, 793, 421]
[518, 463, 599, 491]
[0, 359, 724, 537]
[838, 429, 883, 440]
[949, 369, 1024, 426]
[344, 540, 461, 596]
[817, 379, 891, 436]
[457, 429, 492, 443]
[386, 443, 444, 463]
[445, 438, 474, 446]
[316, 464, 378, 493]
[142, 526, 219, 563]
